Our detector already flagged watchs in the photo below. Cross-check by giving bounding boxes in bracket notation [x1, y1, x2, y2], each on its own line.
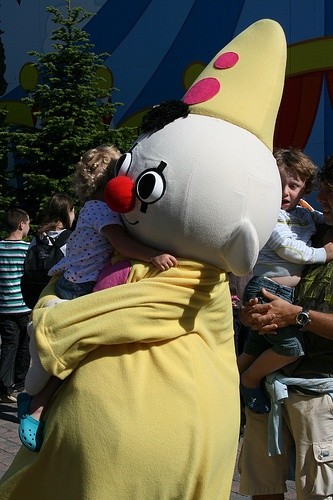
[295, 307, 312, 330]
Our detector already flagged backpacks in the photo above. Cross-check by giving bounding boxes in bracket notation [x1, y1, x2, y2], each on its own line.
[20, 229, 73, 310]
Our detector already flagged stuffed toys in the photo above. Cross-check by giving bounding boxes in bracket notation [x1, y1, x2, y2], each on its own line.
[0, 18, 287, 500]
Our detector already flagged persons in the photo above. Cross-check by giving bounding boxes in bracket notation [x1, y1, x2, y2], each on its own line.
[20, 194, 76, 322]
[0, 209, 33, 402]
[17, 143, 178, 452]
[243, 153, 333, 500]
[234, 148, 333, 414]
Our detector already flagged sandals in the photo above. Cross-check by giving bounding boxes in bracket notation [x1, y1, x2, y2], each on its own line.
[240, 381, 270, 413]
[16, 391, 43, 452]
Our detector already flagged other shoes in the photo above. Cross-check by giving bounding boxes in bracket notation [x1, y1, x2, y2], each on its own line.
[0, 394, 18, 403]
